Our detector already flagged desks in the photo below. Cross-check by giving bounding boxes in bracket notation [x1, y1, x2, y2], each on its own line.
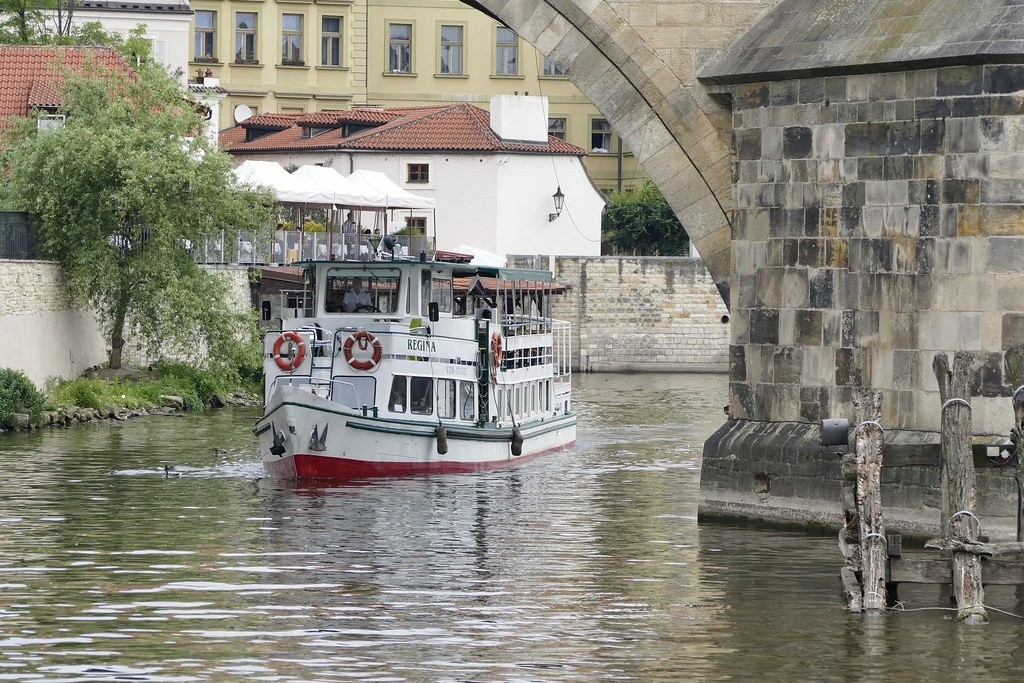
[181, 240, 370, 263]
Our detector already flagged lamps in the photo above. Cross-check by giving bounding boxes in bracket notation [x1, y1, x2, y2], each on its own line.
[549, 185, 565, 222]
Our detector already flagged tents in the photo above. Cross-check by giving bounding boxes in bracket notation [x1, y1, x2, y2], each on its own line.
[221, 160, 438, 266]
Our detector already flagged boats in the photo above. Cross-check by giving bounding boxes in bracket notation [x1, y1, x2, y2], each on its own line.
[242, 251, 579, 492]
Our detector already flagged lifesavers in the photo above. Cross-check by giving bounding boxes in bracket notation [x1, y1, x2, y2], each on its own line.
[491, 330, 503, 367]
[344, 332, 383, 371]
[272, 332, 307, 371]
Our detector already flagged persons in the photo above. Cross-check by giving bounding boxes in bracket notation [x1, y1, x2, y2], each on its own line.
[343, 276, 372, 315]
[342, 211, 381, 260]
[275, 224, 310, 261]
